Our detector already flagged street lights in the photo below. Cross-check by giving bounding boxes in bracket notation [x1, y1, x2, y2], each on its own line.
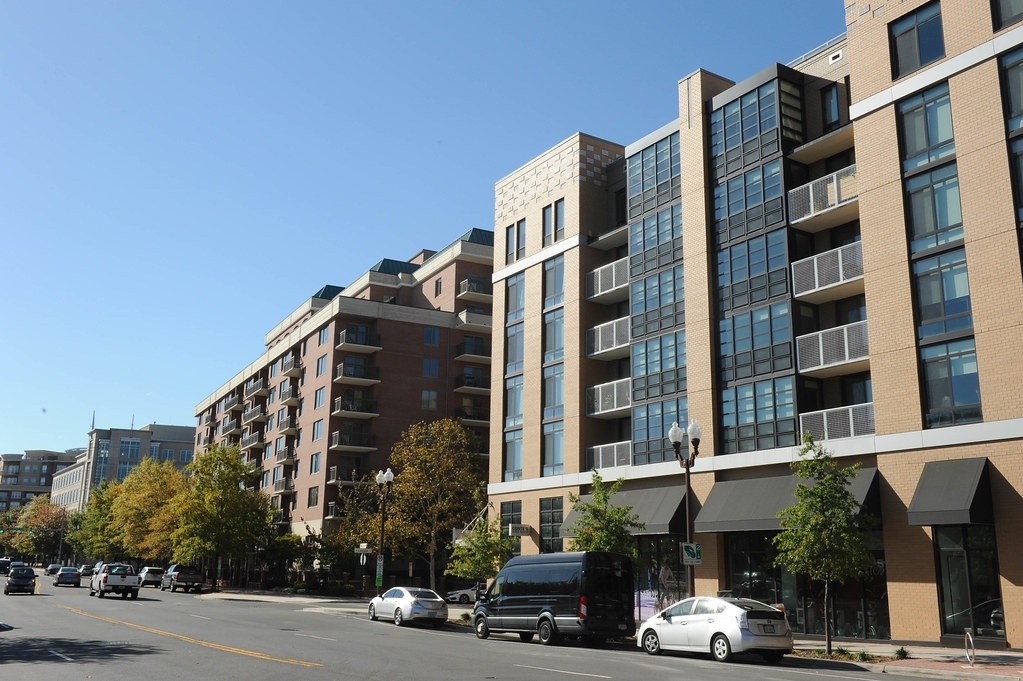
[669, 418, 701, 598]
[375, 467, 394, 599]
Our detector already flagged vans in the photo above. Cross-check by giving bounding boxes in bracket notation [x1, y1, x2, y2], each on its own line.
[471, 550, 638, 646]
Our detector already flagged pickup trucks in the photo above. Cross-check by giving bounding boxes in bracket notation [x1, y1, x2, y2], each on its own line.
[90, 563, 140, 600]
[160, 563, 206, 593]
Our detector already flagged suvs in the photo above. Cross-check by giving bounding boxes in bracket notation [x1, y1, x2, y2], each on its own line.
[138, 567, 165, 589]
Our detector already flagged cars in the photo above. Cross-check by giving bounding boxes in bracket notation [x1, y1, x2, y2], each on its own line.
[44, 564, 62, 576]
[53, 567, 81, 587]
[0, 559, 11, 574]
[78, 565, 91, 575]
[368, 586, 449, 626]
[444, 581, 487, 604]
[637, 595, 793, 662]
[3, 567, 39, 595]
[9, 562, 25, 574]
[92, 560, 103, 575]
